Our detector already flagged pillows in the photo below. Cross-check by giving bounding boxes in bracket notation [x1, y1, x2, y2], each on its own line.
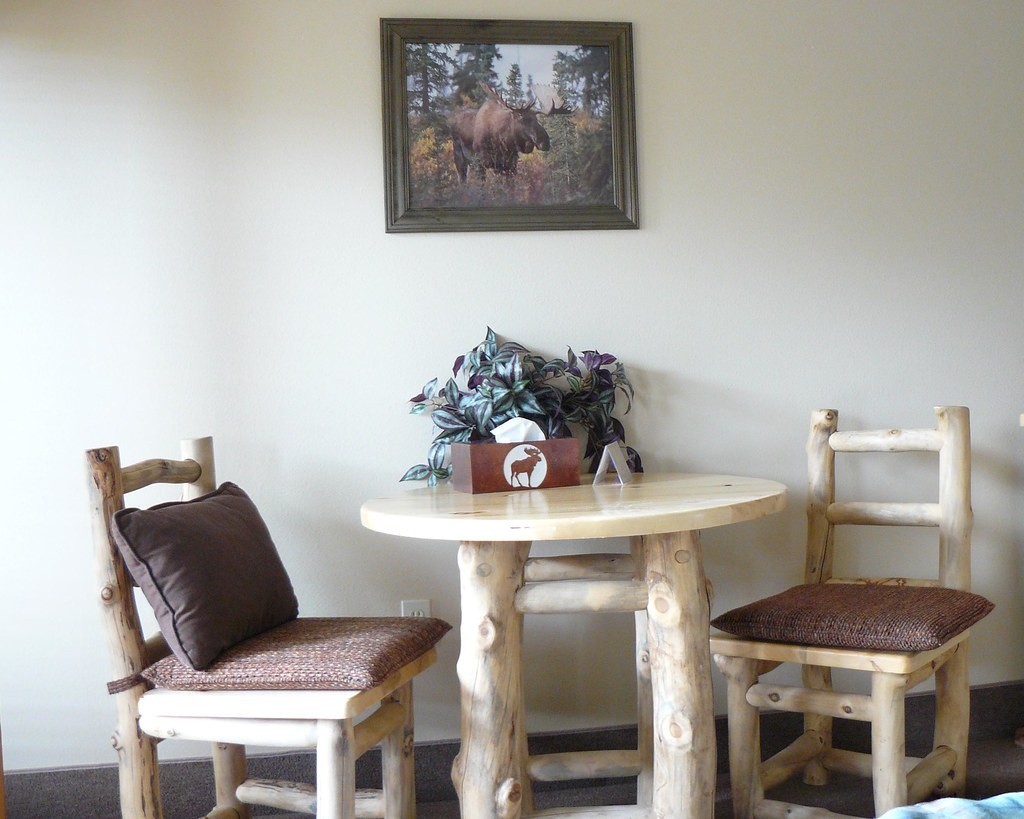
[114, 479, 300, 670]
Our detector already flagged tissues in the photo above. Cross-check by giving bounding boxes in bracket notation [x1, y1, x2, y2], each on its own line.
[450, 417, 581, 495]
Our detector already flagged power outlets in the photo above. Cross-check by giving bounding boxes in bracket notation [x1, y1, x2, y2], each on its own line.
[399, 600, 430, 616]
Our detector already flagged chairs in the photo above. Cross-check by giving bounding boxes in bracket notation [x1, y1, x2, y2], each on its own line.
[83, 435, 452, 819]
[710, 405, 977, 819]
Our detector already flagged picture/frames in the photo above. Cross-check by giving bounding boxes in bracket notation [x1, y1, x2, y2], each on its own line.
[378, 17, 638, 234]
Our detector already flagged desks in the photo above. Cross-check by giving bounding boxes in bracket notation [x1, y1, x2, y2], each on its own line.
[355, 474, 790, 819]
